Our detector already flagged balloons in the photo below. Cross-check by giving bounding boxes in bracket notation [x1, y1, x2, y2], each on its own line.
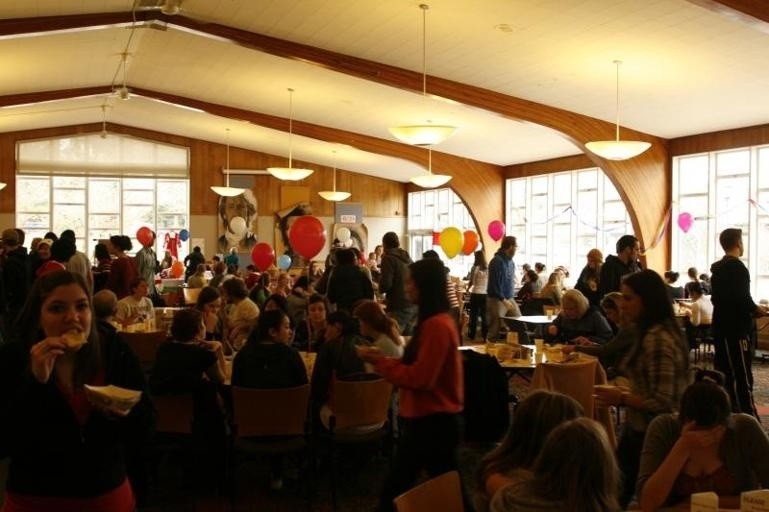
[289, 216, 326, 258]
[486, 219, 505, 242]
[677, 211, 693, 233]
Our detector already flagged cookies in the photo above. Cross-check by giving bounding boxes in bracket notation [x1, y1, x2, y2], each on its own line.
[63, 333, 88, 347]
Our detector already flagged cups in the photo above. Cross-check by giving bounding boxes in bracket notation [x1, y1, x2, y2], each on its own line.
[109, 318, 157, 333]
[487, 331, 546, 364]
[546, 309, 553, 320]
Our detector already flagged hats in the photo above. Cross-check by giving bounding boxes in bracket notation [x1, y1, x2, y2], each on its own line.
[36, 238, 54, 248]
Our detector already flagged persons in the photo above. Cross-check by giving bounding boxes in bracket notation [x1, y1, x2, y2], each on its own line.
[217, 193, 258, 252]
[277, 205, 313, 267]
[1, 227, 769, 511]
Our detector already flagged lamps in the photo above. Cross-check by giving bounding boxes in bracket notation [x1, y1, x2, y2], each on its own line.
[584, 56, 659, 165]
[393, 3, 465, 190]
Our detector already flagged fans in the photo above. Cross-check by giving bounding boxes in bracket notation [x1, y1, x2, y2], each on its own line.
[210, 87, 352, 203]
[79, 0, 206, 142]
